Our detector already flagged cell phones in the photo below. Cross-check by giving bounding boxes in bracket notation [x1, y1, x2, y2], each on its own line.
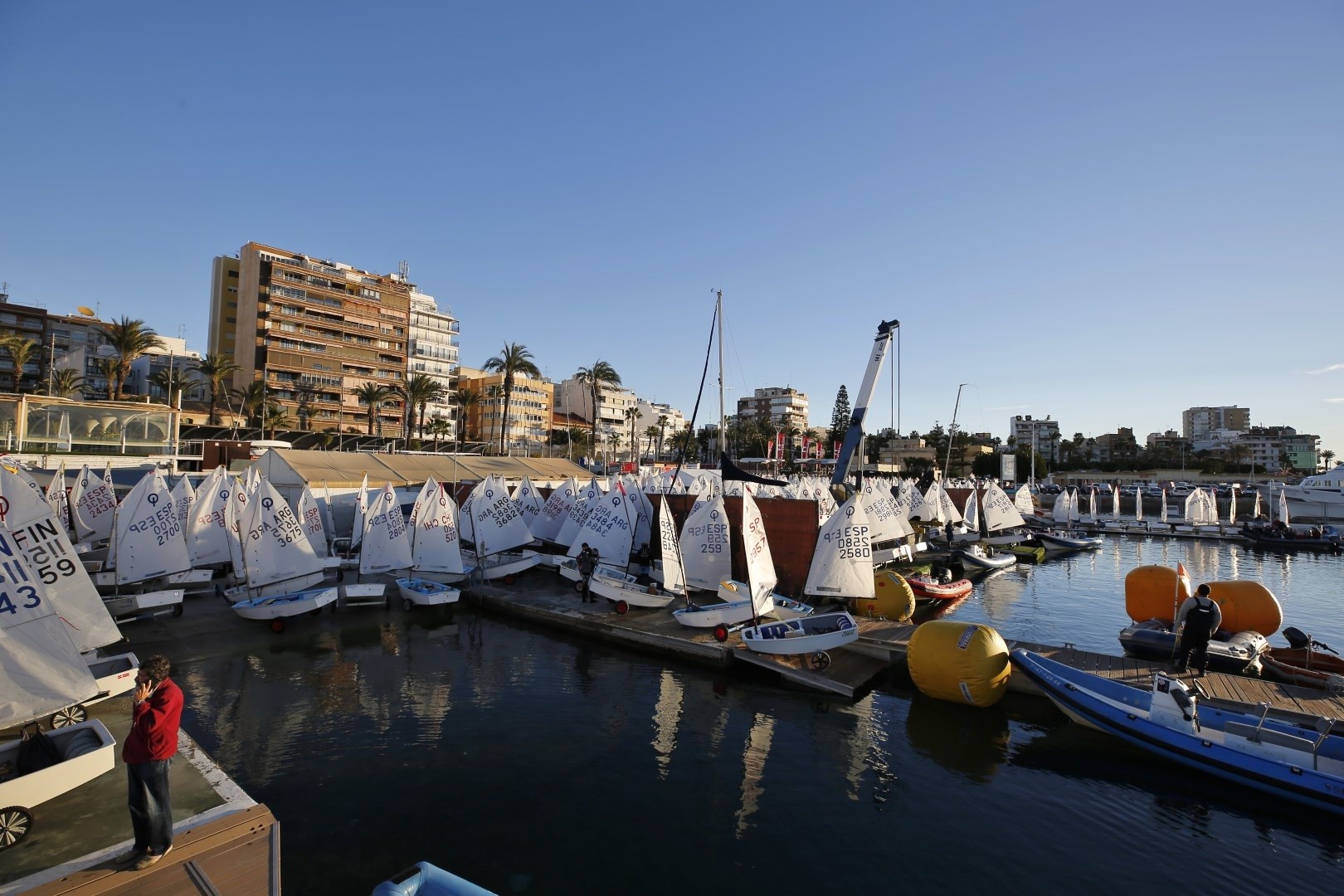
[139, 676, 162, 692]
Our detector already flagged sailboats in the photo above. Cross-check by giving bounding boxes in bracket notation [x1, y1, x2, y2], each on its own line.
[0, 476, 1056, 671]
[1050, 488, 1291, 535]
[0, 468, 141, 850]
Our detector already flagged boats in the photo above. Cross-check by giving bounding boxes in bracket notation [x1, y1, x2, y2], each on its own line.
[1248, 461, 1344, 529]
[905, 533, 1103, 600]
[1008, 646, 1344, 816]
[1119, 619, 1344, 695]
[370, 861, 499, 896]
[1239, 527, 1339, 550]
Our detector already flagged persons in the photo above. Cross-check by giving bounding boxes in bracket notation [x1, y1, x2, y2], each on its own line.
[116, 652, 185, 869]
[637, 541, 655, 574]
[1176, 584, 1222, 677]
[576, 542, 599, 603]
[944, 520, 955, 548]
[1242, 517, 1327, 540]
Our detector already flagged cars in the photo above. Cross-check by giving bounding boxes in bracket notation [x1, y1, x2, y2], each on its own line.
[1033, 481, 1260, 497]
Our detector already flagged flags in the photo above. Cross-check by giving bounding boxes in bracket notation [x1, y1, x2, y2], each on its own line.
[1178, 562, 1191, 581]
[766, 433, 842, 469]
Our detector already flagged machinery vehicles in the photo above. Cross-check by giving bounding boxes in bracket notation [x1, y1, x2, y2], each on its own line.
[828, 319, 900, 507]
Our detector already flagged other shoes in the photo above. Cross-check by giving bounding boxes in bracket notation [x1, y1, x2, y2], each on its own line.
[1199, 672, 1207, 677]
[582, 598, 586, 603]
[590, 599, 598, 603]
[1171, 664, 1187, 672]
[134, 845, 173, 870]
[115, 847, 149, 864]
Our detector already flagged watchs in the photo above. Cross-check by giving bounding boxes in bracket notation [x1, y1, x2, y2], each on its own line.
[136, 700, 145, 706]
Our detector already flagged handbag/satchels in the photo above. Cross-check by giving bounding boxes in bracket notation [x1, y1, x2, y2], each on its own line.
[17, 721, 61, 775]
